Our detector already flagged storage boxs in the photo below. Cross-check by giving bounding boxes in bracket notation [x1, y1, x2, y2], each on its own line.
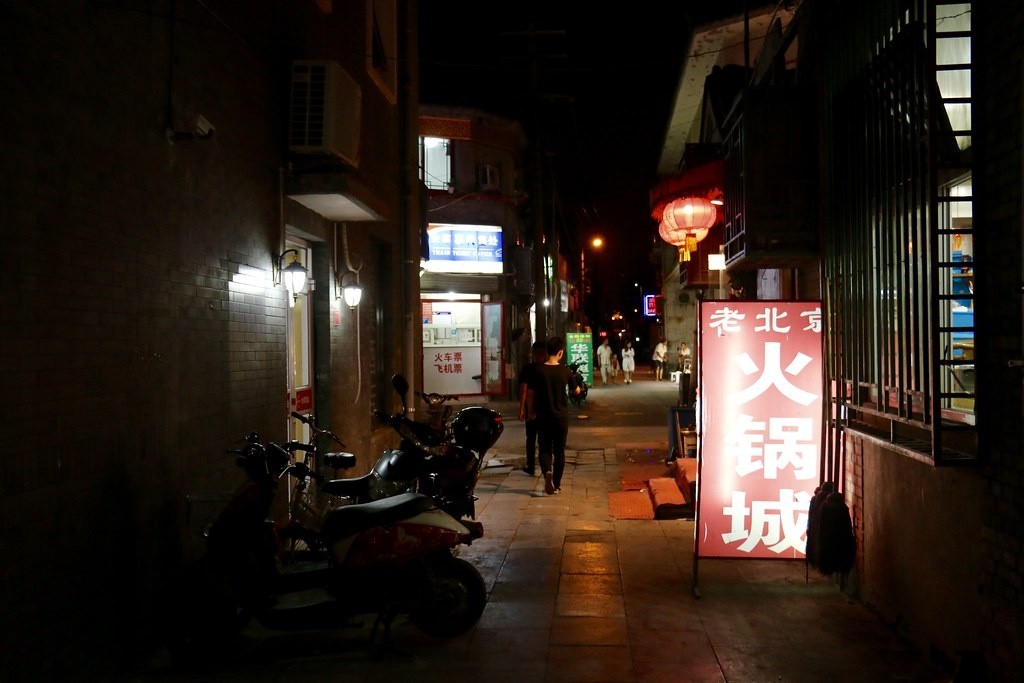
[451, 407, 505, 449]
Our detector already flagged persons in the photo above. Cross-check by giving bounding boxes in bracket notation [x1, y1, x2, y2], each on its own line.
[620, 340, 635, 383]
[518, 341, 547, 477]
[652, 336, 668, 381]
[609, 354, 620, 382]
[678, 342, 691, 370]
[526, 337, 581, 495]
[597, 336, 613, 387]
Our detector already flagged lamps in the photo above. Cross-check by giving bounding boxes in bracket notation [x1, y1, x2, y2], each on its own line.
[334, 270, 363, 313]
[271, 249, 308, 303]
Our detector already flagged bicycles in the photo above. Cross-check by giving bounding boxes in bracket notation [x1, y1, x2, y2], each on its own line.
[284, 410, 362, 561]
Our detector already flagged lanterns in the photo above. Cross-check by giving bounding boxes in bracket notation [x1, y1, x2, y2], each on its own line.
[658, 218, 709, 263]
[662, 196, 717, 252]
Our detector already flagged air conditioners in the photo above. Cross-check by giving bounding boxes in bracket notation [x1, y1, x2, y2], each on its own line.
[285, 58, 364, 172]
[479, 163, 500, 190]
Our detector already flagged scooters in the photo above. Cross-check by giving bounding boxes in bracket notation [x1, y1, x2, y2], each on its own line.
[162, 431, 489, 679]
[563, 356, 588, 407]
[357, 373, 505, 524]
[412, 390, 459, 437]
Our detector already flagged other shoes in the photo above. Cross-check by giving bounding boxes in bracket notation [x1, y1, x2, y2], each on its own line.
[603, 382, 618, 386]
[629, 378, 633, 383]
[624, 379, 628, 384]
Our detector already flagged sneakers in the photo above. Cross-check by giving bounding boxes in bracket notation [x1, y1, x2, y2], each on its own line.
[544, 471, 555, 495]
[554, 488, 563, 493]
[522, 465, 533, 475]
